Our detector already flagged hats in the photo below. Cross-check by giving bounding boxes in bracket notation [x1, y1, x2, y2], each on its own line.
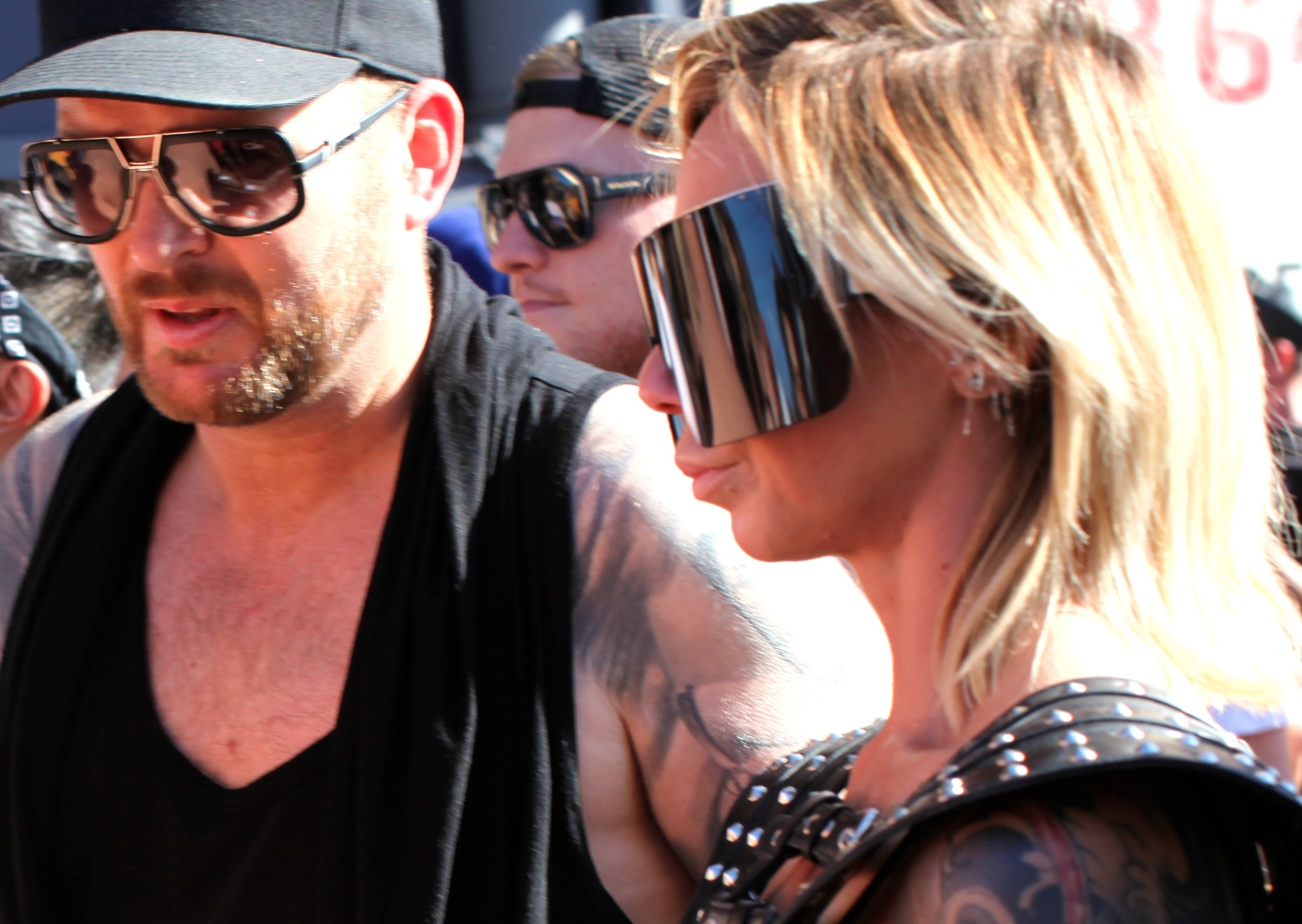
[514, 11, 712, 153]
[1, 1, 445, 110]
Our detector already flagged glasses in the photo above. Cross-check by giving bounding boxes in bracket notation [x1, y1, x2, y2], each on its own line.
[471, 167, 675, 250]
[630, 177, 992, 449]
[15, 84, 409, 242]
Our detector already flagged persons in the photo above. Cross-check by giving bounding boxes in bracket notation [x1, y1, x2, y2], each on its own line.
[482, 12, 706, 371]
[0, 1, 1302, 924]
